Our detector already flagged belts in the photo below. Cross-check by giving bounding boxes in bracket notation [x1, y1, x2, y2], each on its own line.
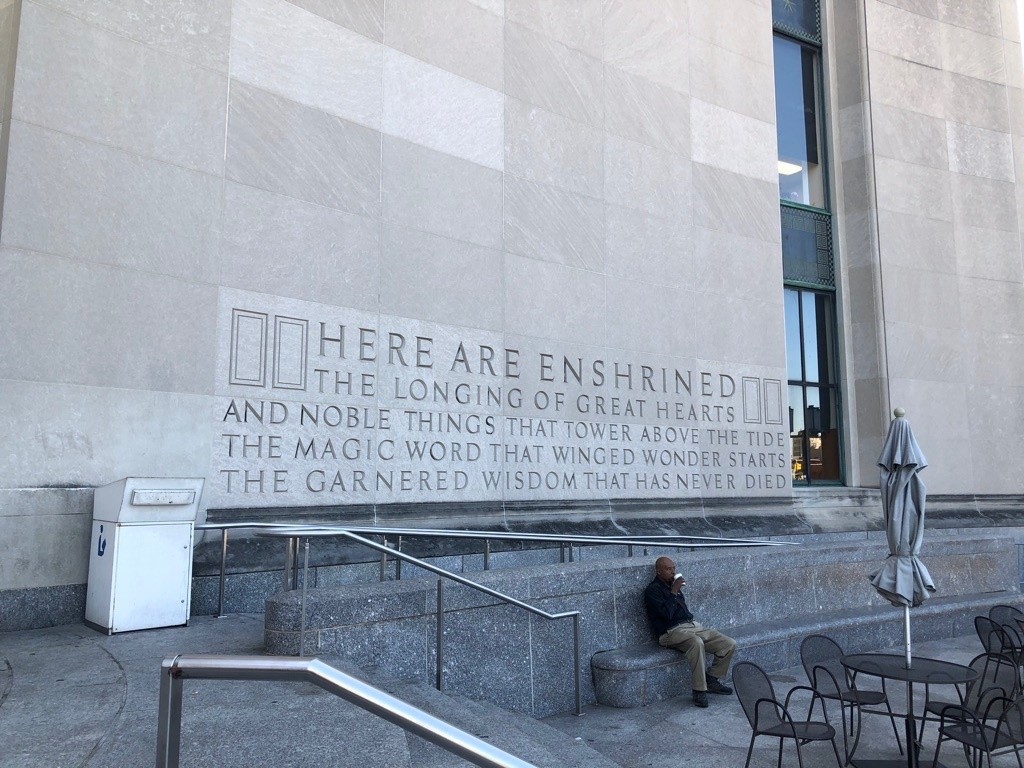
[669, 618, 693, 630]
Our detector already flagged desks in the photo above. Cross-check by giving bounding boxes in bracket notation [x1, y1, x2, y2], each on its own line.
[839, 651, 979, 768]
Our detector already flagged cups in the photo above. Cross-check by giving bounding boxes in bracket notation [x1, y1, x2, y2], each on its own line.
[674, 573, 686, 586]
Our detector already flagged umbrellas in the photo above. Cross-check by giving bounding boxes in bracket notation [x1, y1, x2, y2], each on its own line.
[867, 407, 936, 670]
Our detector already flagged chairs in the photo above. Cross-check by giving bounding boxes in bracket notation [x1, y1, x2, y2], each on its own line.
[731, 661, 843, 768]
[925, 605, 1024, 768]
[796, 634, 904, 763]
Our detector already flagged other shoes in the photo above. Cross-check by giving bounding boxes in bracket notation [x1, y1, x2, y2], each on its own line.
[692, 689, 708, 707]
[706, 679, 733, 695]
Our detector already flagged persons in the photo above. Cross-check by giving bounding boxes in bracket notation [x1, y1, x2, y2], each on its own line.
[646, 557, 736, 708]
